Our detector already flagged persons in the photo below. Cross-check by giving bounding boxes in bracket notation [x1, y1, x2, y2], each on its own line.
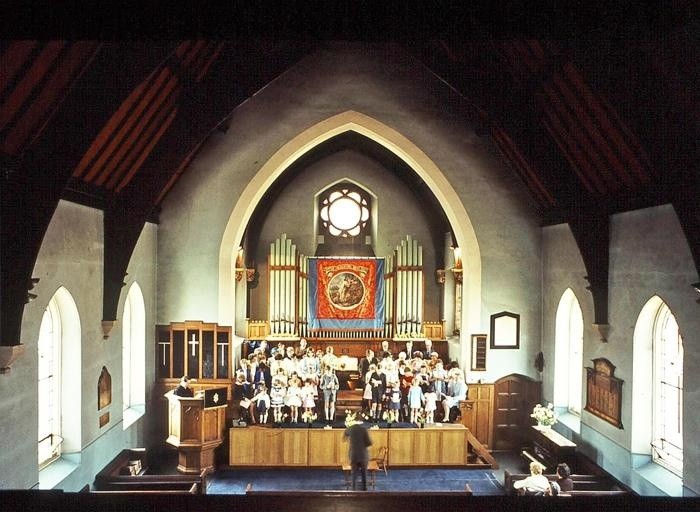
[513, 462, 552, 497]
[343, 415, 373, 490]
[360, 339, 469, 424]
[174, 376, 193, 397]
[338, 363, 352, 390]
[555, 462, 574, 491]
[535, 481, 561, 496]
[342, 280, 358, 307]
[232, 336, 339, 426]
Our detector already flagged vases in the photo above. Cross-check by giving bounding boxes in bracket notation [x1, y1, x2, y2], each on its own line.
[538, 424, 552, 432]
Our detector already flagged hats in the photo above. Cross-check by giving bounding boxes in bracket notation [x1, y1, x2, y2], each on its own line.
[271, 347, 280, 353]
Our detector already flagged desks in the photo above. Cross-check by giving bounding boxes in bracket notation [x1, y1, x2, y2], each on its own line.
[342, 460, 378, 490]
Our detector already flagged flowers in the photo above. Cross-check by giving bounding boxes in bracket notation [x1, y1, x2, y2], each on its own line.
[530, 402, 559, 426]
[382, 408, 399, 423]
[302, 411, 318, 422]
[344, 408, 369, 421]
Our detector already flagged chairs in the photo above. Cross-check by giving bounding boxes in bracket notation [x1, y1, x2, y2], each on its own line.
[370, 446, 388, 477]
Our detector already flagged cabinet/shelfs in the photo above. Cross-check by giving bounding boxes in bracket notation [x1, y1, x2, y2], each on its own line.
[529, 425, 577, 472]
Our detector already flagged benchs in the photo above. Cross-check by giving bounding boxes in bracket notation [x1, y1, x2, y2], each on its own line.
[504, 471, 627, 497]
[81, 447, 207, 495]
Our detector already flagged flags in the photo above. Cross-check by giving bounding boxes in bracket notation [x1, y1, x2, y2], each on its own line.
[304, 256, 386, 337]
